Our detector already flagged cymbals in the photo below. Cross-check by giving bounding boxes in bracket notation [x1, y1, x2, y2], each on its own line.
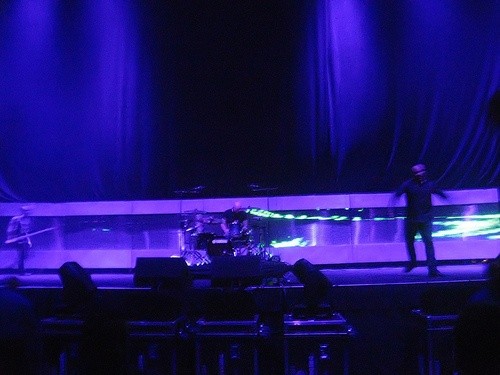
[250, 184, 279, 193]
[175, 186, 200, 194]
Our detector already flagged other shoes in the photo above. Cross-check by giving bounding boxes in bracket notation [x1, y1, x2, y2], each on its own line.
[403, 262, 416, 272]
[428, 269, 443, 277]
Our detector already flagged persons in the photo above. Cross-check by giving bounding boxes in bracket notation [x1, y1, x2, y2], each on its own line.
[393, 164, 447, 277]
[8, 206, 32, 276]
[0, 276, 37, 375]
[454, 253, 500, 375]
[220, 201, 247, 236]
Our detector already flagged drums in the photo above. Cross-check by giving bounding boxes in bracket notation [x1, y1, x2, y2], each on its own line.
[206, 240, 232, 258]
[191, 232, 208, 248]
